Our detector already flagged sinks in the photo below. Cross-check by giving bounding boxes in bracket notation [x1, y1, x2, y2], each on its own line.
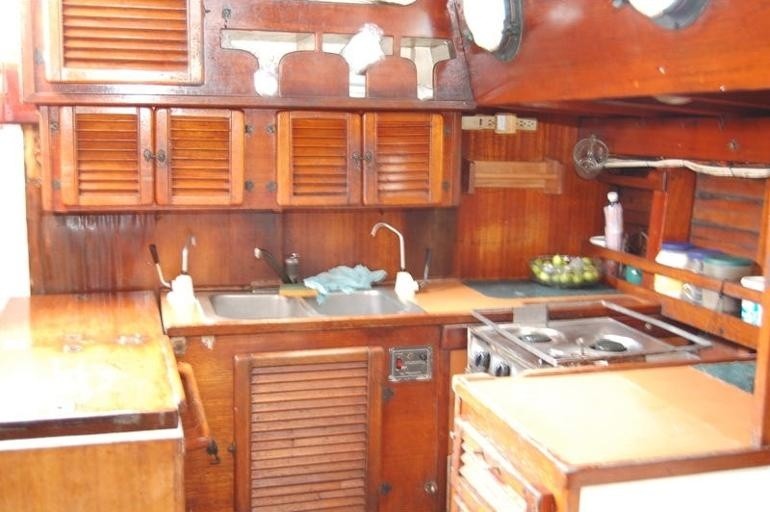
[195, 290, 319, 325]
[303, 284, 428, 318]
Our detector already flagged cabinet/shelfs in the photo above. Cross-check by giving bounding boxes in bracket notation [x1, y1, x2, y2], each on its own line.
[273, 110, 445, 212]
[58, 104, 245, 212]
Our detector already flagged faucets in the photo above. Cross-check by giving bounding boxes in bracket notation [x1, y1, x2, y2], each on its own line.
[369, 222, 418, 301]
[254, 245, 301, 283]
[169, 228, 198, 300]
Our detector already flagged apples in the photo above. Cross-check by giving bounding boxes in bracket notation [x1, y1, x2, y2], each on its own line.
[532, 254, 596, 285]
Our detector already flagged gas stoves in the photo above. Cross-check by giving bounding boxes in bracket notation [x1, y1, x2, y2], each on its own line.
[463, 315, 701, 377]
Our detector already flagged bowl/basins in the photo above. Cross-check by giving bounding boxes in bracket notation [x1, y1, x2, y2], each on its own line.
[526, 252, 606, 289]
